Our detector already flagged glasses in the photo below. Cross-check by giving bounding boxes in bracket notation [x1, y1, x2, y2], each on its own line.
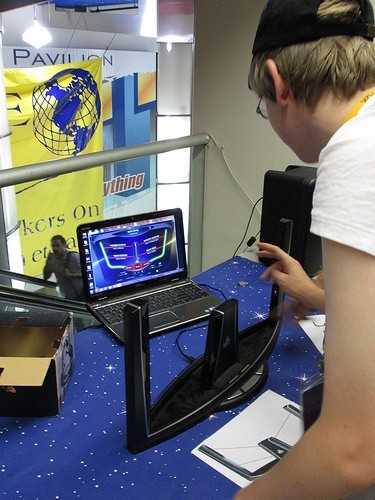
[253, 74, 274, 120]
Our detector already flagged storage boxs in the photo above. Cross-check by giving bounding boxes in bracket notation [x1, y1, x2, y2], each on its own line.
[0, 310, 74, 417]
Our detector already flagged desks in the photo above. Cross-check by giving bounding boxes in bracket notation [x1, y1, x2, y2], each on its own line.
[0, 257, 325, 500]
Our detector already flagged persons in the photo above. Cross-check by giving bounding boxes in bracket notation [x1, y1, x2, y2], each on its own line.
[42, 235, 83, 301]
[229, 0, 375, 500]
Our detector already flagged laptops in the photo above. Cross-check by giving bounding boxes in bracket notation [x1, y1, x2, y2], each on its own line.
[79, 208, 226, 346]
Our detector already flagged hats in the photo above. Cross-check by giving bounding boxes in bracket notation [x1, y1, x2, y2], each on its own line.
[252, 0, 375, 54]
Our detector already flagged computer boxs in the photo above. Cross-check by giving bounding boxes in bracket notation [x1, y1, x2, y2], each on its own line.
[258, 169, 323, 276]
